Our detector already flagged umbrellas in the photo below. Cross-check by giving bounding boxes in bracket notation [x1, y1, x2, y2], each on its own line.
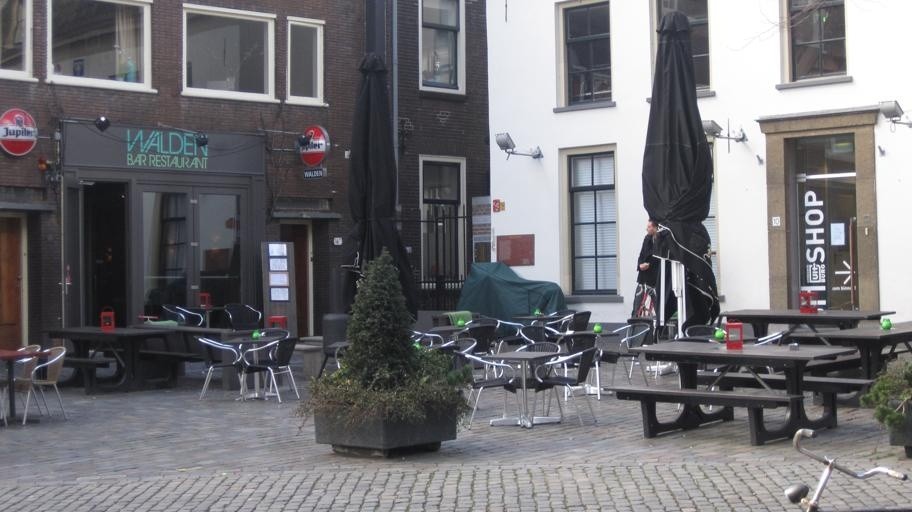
[343, 42, 424, 328]
[628, 8, 721, 328]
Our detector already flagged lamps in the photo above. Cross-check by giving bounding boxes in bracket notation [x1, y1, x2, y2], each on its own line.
[878, 101, 912, 130]
[155, 121, 210, 148]
[698, 120, 749, 144]
[493, 132, 544, 164]
[50, 113, 111, 134]
[256, 127, 309, 148]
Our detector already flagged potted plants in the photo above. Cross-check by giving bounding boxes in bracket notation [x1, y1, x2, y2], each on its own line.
[287, 241, 470, 456]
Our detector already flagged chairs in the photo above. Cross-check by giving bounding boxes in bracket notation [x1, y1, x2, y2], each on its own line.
[2, 294, 300, 427]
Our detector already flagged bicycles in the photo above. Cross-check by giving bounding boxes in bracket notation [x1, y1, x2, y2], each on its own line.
[785, 427, 912, 512]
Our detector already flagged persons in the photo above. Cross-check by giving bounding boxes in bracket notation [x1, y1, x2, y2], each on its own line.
[629, 216, 660, 330]
